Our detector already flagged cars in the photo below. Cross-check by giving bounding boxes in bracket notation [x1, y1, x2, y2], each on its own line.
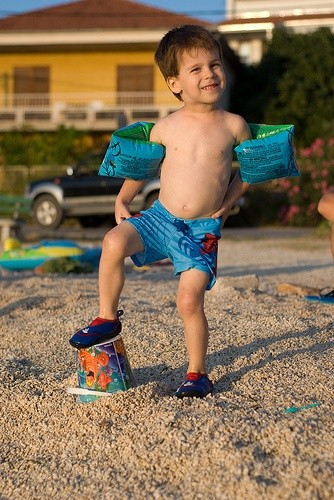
[28, 139, 291, 231]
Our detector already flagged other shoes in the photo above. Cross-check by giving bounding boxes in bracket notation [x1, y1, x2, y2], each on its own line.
[68, 310, 123, 349]
[175, 371, 213, 399]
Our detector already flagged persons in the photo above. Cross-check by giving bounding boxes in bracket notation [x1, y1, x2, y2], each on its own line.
[69, 26, 301, 400]
[306, 191, 334, 304]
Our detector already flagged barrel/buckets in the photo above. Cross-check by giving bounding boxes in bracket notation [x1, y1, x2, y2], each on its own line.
[67, 334, 138, 403]
